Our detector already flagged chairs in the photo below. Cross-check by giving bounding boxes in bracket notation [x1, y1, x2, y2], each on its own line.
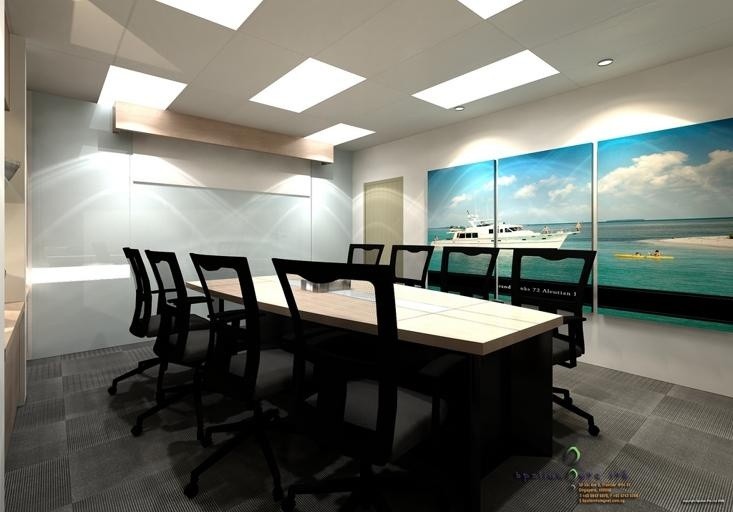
[108, 242, 599, 511]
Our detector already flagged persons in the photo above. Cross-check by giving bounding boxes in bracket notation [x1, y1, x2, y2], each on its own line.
[544, 226, 548, 234]
[576, 223, 581, 231]
[654, 250, 660, 256]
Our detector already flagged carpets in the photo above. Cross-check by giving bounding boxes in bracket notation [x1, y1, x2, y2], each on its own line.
[5, 337, 732, 512]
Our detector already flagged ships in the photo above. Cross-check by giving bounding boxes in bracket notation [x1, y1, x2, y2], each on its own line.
[431, 210, 579, 252]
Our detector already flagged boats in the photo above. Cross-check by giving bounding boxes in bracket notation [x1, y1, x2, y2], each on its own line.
[615, 251, 673, 262]
